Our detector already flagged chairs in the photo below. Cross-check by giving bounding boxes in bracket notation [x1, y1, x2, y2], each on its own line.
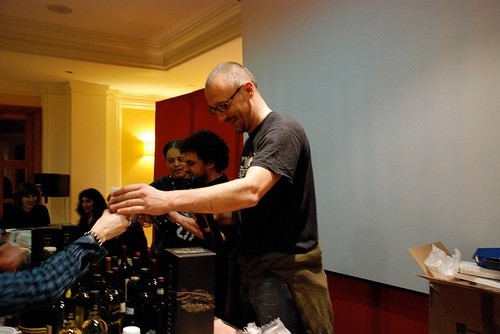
[17, 324, 52, 334]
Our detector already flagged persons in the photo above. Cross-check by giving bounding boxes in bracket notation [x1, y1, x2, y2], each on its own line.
[109, 62, 335, 334]
[0, 129, 238, 334]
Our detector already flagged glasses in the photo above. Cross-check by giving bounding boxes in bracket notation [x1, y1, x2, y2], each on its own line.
[208, 84, 245, 115]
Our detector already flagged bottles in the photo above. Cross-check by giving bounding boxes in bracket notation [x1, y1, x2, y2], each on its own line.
[16, 246, 167, 334]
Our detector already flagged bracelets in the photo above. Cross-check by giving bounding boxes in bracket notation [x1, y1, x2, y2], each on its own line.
[84, 231, 103, 246]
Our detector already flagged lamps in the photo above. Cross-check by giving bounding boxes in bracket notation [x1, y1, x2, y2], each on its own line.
[137, 127, 156, 165]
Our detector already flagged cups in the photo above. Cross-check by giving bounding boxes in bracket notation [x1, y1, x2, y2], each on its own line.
[110, 186, 122, 212]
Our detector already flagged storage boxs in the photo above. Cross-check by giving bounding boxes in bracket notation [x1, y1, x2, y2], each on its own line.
[158, 244, 219, 334]
[407, 240, 500, 334]
